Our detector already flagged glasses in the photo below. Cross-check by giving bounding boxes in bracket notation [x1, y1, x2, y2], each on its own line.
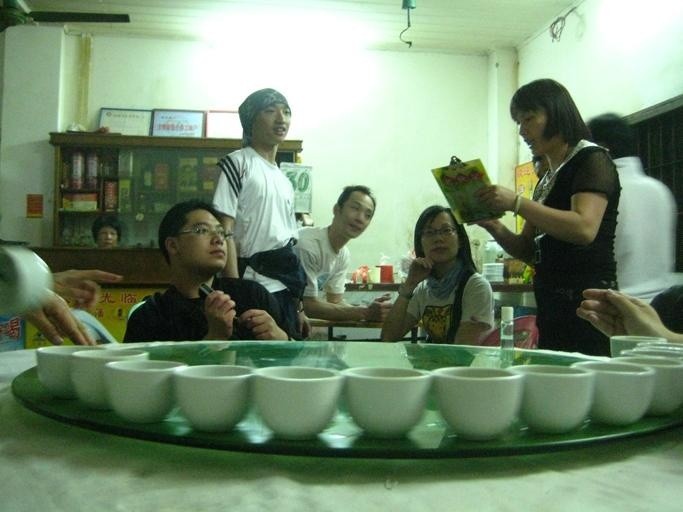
[175, 226, 233, 241]
[421, 226, 457, 240]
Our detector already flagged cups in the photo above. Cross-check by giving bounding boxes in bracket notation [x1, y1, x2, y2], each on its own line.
[573, 361, 656, 428]
[609, 335, 683, 416]
[507, 364, 594, 435]
[367, 267, 380, 284]
[250, 364, 343, 438]
[105, 358, 187, 428]
[174, 364, 250, 438]
[342, 367, 431, 438]
[38, 347, 108, 401]
[68, 347, 151, 413]
[431, 367, 526, 437]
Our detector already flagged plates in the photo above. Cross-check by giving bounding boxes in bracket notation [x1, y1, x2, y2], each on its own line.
[483, 263, 504, 282]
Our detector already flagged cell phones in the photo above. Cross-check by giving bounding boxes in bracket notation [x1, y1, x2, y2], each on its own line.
[198, 283, 238, 322]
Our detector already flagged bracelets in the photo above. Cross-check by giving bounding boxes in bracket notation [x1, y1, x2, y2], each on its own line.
[398, 285, 413, 299]
[512, 192, 522, 218]
[286, 332, 292, 342]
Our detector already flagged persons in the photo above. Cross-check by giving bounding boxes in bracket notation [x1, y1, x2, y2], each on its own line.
[531, 153, 549, 180]
[92, 214, 124, 248]
[379, 205, 496, 346]
[586, 113, 679, 289]
[295, 186, 394, 324]
[212, 88, 314, 341]
[1, 245, 125, 346]
[467, 77, 623, 356]
[124, 199, 294, 341]
[576, 280, 683, 361]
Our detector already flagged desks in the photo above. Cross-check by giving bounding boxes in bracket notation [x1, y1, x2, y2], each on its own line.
[346, 279, 534, 342]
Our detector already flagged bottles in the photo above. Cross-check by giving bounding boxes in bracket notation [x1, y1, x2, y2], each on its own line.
[59, 145, 174, 213]
[498, 308, 515, 351]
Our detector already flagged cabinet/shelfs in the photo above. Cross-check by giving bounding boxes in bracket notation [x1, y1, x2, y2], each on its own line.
[48, 131, 303, 245]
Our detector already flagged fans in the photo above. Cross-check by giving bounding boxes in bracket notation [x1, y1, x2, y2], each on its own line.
[1, 1, 131, 38]
